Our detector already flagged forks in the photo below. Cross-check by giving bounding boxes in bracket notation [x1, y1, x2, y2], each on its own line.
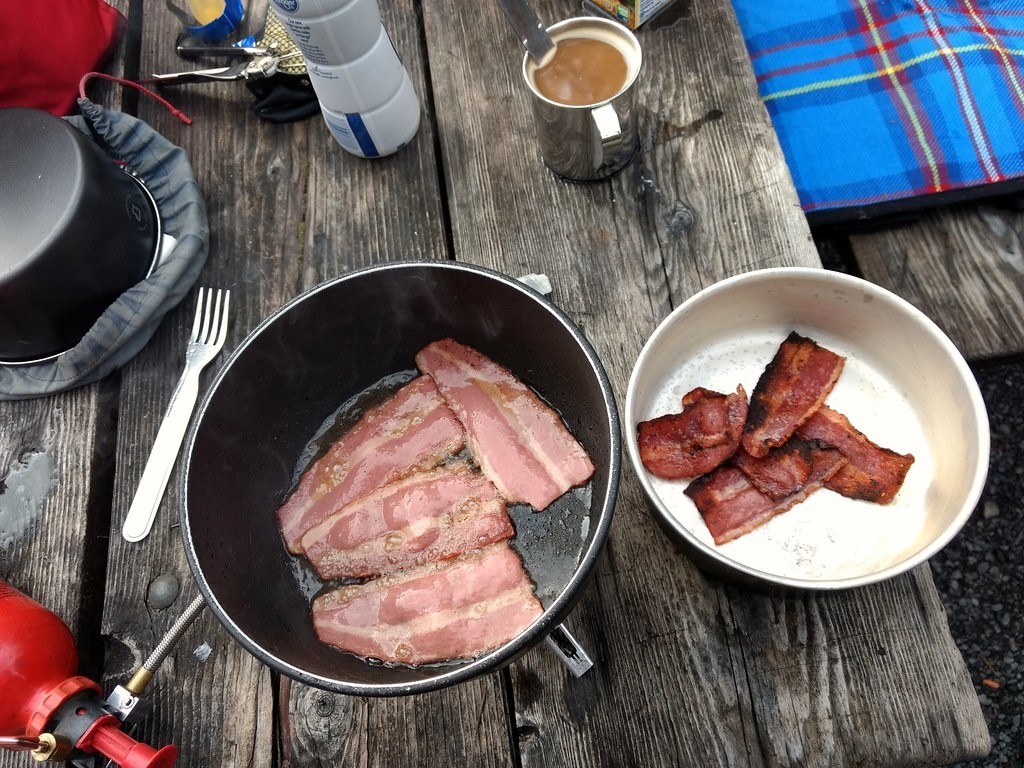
[122, 287, 232, 544]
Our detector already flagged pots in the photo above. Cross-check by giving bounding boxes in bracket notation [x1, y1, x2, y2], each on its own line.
[180, 257, 621, 698]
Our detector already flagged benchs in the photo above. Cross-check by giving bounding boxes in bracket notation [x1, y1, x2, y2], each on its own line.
[731, 0, 1024, 364]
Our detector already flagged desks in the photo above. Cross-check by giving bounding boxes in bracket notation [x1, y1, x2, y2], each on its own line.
[0, 0, 991, 768]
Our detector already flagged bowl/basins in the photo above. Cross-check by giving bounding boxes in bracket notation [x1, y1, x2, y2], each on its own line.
[0, 105, 162, 366]
[625, 265, 992, 588]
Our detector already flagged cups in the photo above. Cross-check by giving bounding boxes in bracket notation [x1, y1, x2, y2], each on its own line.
[523, 15, 642, 184]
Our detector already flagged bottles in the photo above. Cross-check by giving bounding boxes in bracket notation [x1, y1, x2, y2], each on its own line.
[266, 0, 420, 157]
